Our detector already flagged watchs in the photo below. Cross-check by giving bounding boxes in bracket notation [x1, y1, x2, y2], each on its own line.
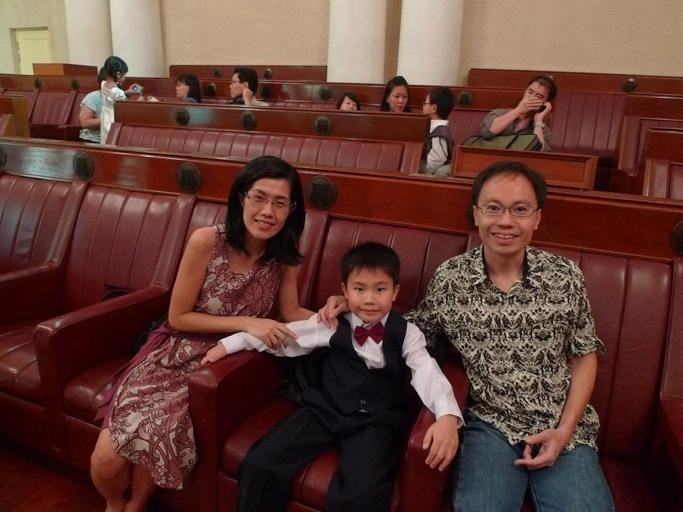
[532, 124, 547, 130]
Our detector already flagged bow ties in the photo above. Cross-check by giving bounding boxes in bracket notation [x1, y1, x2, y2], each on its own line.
[355, 322, 384, 346]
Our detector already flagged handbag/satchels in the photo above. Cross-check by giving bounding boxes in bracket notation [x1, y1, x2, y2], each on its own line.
[461, 135, 542, 151]
[130, 321, 164, 355]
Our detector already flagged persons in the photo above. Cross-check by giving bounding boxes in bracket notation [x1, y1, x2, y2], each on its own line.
[477, 77, 557, 153]
[380, 77, 415, 113]
[202, 241, 465, 510]
[89, 155, 319, 511]
[318, 164, 619, 510]
[224, 68, 270, 109]
[335, 91, 361, 111]
[99, 56, 144, 147]
[422, 86, 454, 177]
[148, 72, 201, 104]
[79, 66, 104, 145]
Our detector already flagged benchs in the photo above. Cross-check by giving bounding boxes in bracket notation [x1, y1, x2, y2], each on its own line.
[0, 72, 683, 512]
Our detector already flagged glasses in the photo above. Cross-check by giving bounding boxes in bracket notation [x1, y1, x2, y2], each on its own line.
[232, 79, 240, 84]
[244, 192, 295, 209]
[423, 101, 433, 106]
[475, 204, 538, 216]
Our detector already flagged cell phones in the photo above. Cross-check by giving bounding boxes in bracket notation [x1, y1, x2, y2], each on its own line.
[133, 85, 144, 96]
[538, 105, 547, 112]
